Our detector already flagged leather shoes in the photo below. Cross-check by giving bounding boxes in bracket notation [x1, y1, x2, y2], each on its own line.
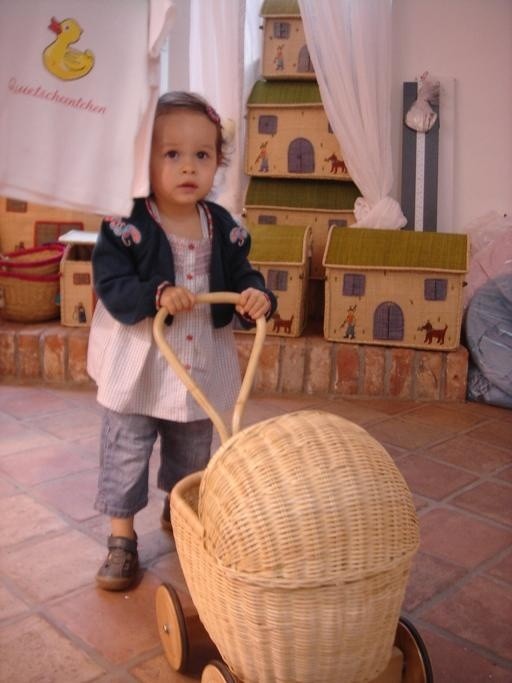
[160, 503, 171, 530]
[95, 532, 138, 592]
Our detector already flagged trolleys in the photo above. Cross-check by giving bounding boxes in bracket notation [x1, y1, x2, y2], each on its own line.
[154, 288, 436, 683]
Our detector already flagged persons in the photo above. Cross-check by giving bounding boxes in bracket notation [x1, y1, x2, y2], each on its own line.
[85, 91, 277, 590]
[273, 43, 286, 72]
[253, 141, 270, 172]
[340, 304, 358, 338]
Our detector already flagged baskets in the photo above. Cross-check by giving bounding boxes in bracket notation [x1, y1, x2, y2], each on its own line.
[0, 243, 63, 324]
